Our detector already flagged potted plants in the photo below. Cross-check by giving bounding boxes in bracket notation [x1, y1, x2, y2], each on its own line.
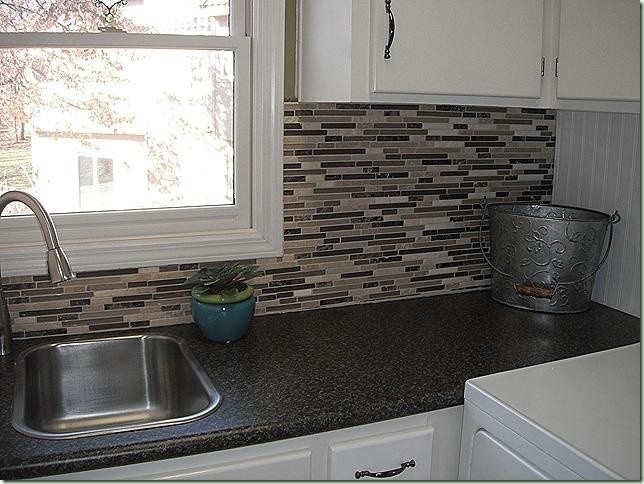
[182, 262, 267, 344]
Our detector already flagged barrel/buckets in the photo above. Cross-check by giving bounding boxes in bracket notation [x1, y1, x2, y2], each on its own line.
[479, 197, 622, 315]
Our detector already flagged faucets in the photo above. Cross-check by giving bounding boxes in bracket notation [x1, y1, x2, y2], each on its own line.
[0, 191, 75, 356]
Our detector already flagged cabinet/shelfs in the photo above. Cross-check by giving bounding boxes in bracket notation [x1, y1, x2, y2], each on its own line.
[371, 0, 545, 101]
[189, 417, 435, 481]
[555, 1, 643, 104]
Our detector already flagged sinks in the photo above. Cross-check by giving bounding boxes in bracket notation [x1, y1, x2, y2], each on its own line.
[12, 332, 224, 440]
